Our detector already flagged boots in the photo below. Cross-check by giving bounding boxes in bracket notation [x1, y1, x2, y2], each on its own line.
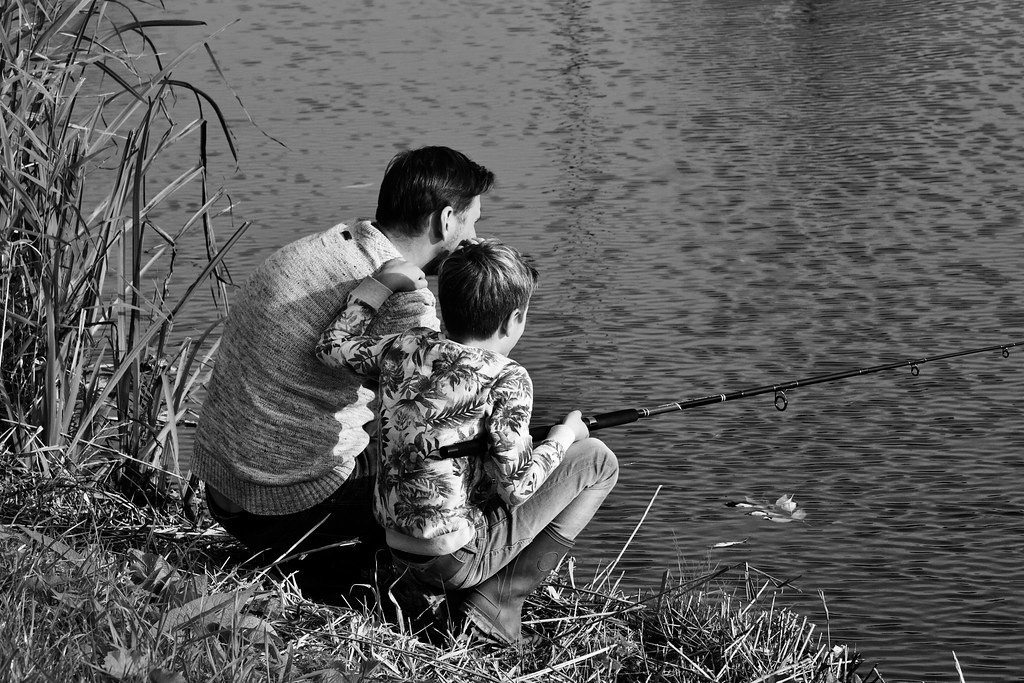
[459, 525, 573, 653]
[413, 588, 468, 643]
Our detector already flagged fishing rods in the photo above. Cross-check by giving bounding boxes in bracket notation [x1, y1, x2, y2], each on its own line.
[439, 339, 1023, 458]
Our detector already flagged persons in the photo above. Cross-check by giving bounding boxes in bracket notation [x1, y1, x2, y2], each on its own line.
[315, 237, 619, 648]
[189, 146, 496, 561]
